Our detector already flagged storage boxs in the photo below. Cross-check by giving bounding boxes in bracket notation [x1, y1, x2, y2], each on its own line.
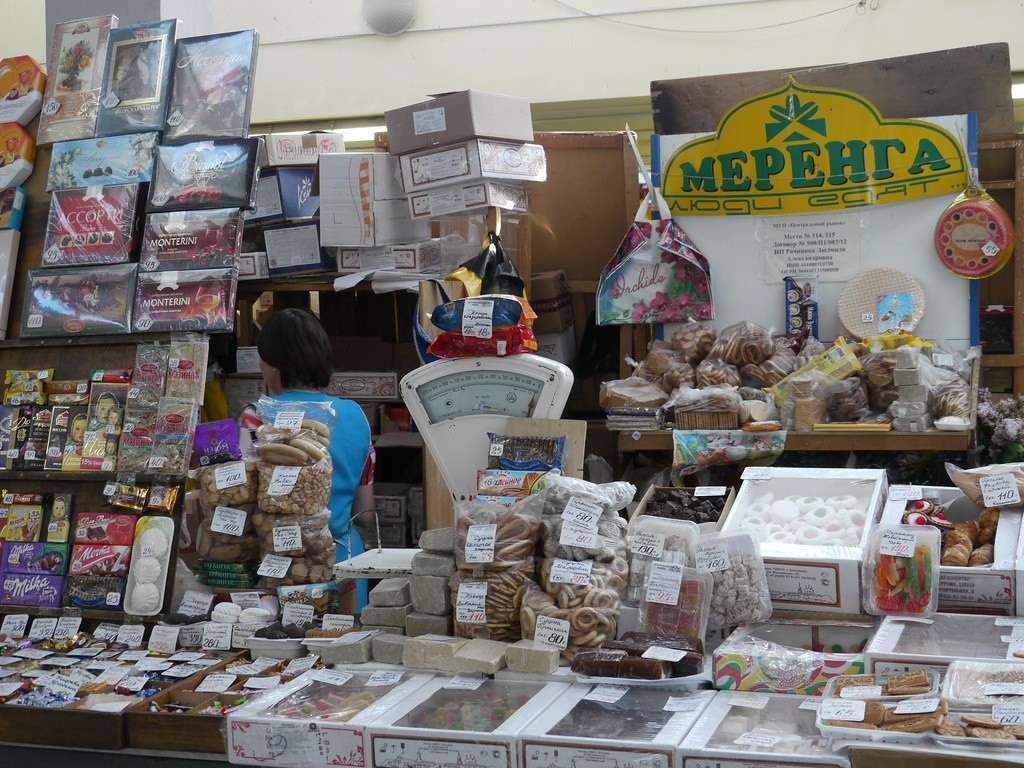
[0, 347, 1024, 767]
[239, 83, 640, 371]
[0, 13, 263, 346]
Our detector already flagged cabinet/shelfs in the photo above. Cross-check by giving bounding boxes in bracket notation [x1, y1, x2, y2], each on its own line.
[219, 277, 423, 451]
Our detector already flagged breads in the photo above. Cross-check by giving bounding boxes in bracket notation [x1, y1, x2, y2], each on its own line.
[197, 419, 332, 584]
[905, 499, 1000, 568]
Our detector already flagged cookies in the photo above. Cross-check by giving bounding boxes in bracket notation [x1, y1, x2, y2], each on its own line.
[452, 510, 628, 665]
[605, 321, 971, 430]
[824, 670, 1024, 739]
